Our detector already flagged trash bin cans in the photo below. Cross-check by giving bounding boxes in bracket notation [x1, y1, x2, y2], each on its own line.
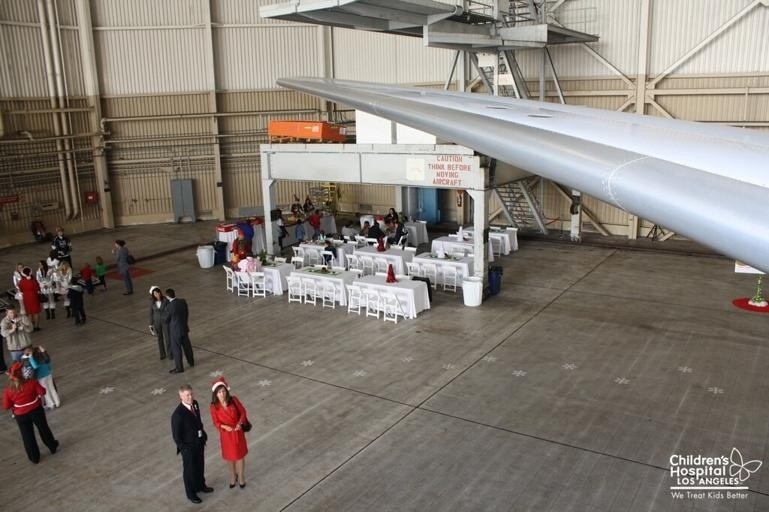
[196, 245, 217, 268]
[208, 241, 228, 265]
[462, 276, 483, 307]
[488, 266, 503, 296]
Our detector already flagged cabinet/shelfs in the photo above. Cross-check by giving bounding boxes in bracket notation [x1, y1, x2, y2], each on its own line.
[169, 177, 195, 222]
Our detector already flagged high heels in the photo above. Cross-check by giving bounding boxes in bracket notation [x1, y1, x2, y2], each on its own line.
[239, 479, 245, 488]
[230, 474, 238, 488]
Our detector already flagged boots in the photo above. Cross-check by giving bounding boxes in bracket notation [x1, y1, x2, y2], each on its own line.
[65, 306, 71, 318]
[50, 309, 56, 319]
[44, 309, 50, 319]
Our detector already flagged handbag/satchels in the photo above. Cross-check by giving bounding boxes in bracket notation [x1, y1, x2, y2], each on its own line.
[242, 419, 251, 432]
[38, 293, 48, 303]
[14, 291, 23, 300]
[127, 256, 136, 265]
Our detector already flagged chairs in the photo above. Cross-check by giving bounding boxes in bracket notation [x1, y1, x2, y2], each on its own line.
[345, 284, 370, 315]
[375, 272, 388, 277]
[490, 236, 505, 257]
[316, 281, 342, 309]
[304, 246, 325, 265]
[404, 247, 418, 256]
[412, 277, 431, 303]
[421, 263, 444, 291]
[290, 256, 304, 268]
[346, 254, 362, 272]
[292, 247, 309, 265]
[365, 237, 380, 248]
[374, 254, 397, 275]
[388, 233, 409, 249]
[355, 235, 366, 244]
[351, 268, 363, 279]
[222, 265, 238, 293]
[377, 290, 410, 324]
[440, 265, 464, 293]
[285, 275, 306, 303]
[249, 271, 278, 298]
[406, 261, 423, 278]
[395, 274, 411, 280]
[362, 287, 396, 318]
[235, 271, 259, 297]
[389, 244, 404, 251]
[274, 256, 287, 264]
[361, 254, 376, 274]
[320, 250, 339, 269]
[302, 277, 323, 306]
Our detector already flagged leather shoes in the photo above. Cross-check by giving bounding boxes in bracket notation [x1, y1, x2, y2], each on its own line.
[169, 368, 184, 374]
[196, 485, 213, 492]
[188, 494, 201, 504]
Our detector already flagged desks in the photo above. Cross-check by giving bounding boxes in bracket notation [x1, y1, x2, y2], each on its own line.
[241, 259, 295, 296]
[216, 217, 279, 263]
[412, 251, 474, 285]
[462, 224, 519, 255]
[360, 214, 430, 245]
[356, 246, 412, 276]
[432, 234, 496, 261]
[353, 273, 431, 320]
[276, 209, 333, 249]
[291, 264, 361, 307]
[299, 239, 353, 267]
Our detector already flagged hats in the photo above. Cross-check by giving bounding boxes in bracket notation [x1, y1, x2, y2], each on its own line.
[7, 306, 15, 309]
[9, 361, 22, 380]
[210, 377, 231, 392]
[149, 286, 161, 295]
[20, 266, 33, 281]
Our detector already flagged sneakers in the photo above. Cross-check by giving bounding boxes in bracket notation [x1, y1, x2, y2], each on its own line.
[169, 356, 173, 360]
[124, 292, 133, 295]
[160, 357, 165, 360]
[34, 328, 42, 331]
[51, 440, 58, 455]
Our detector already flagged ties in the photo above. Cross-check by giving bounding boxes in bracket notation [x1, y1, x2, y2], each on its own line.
[191, 406, 196, 416]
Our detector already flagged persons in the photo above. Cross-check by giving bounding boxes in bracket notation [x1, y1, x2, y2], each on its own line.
[21, 345, 61, 409]
[0, 304, 34, 360]
[80, 262, 96, 294]
[3, 361, 58, 464]
[46, 250, 62, 301]
[111, 239, 133, 295]
[50, 226, 73, 266]
[95, 256, 109, 290]
[271, 196, 341, 266]
[161, 288, 194, 373]
[13, 263, 27, 316]
[67, 277, 87, 323]
[18, 266, 43, 330]
[172, 384, 214, 504]
[341, 207, 408, 246]
[148, 285, 173, 360]
[36, 260, 57, 319]
[0, 336, 7, 373]
[56, 261, 73, 318]
[209, 376, 248, 488]
